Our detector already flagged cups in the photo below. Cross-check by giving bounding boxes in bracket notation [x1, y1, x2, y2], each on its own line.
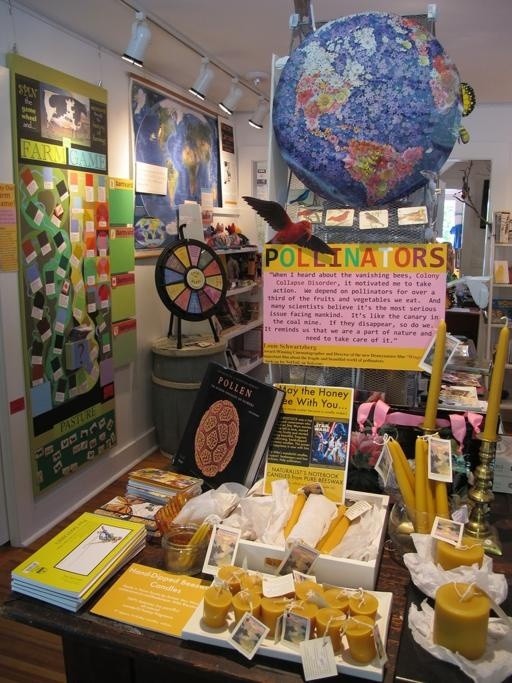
[159, 523, 209, 576]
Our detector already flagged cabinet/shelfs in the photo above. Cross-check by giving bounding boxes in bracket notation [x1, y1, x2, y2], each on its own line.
[176, 204, 512, 425]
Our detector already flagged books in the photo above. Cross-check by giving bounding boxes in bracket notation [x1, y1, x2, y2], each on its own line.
[89, 562, 213, 640]
[10, 466, 205, 614]
[178, 360, 285, 492]
[261, 378, 352, 506]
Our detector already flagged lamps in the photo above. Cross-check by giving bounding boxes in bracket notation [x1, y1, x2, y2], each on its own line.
[120, 11, 270, 129]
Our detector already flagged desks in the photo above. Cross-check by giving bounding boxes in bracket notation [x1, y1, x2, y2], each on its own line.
[0, 466, 512, 683]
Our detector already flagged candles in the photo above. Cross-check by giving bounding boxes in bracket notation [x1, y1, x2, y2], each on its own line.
[231, 588, 261, 633]
[315, 608, 345, 652]
[338, 615, 378, 662]
[311, 499, 362, 561]
[433, 534, 487, 572]
[280, 487, 310, 542]
[347, 588, 379, 619]
[202, 584, 231, 631]
[429, 580, 496, 657]
[215, 563, 244, 590]
[258, 594, 284, 642]
[238, 572, 262, 597]
[286, 600, 319, 646]
[295, 578, 320, 603]
[320, 586, 346, 612]
[379, 433, 455, 537]
[478, 317, 510, 442]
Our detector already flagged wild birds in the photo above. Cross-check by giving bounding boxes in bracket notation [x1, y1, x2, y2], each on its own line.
[291, 187, 313, 205]
[241, 194, 337, 257]
